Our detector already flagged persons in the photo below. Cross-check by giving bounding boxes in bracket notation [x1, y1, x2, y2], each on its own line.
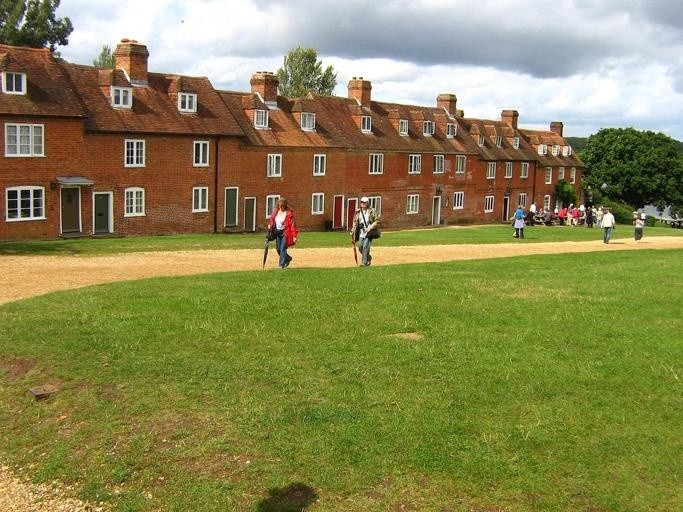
[526, 202, 537, 226]
[633, 215, 645, 241]
[509, 208, 525, 236]
[514, 205, 525, 238]
[351, 197, 380, 266]
[266, 198, 298, 268]
[537, 203, 604, 228]
[600, 208, 615, 244]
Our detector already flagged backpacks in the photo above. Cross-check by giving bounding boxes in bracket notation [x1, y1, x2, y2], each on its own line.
[515, 209, 524, 220]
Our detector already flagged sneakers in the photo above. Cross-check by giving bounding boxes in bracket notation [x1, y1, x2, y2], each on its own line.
[279, 256, 292, 269]
[360, 256, 371, 266]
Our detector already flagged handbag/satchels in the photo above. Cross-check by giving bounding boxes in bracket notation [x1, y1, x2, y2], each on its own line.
[366, 229, 380, 239]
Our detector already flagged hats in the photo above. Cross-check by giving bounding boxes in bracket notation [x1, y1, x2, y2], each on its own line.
[360, 197, 369, 204]
